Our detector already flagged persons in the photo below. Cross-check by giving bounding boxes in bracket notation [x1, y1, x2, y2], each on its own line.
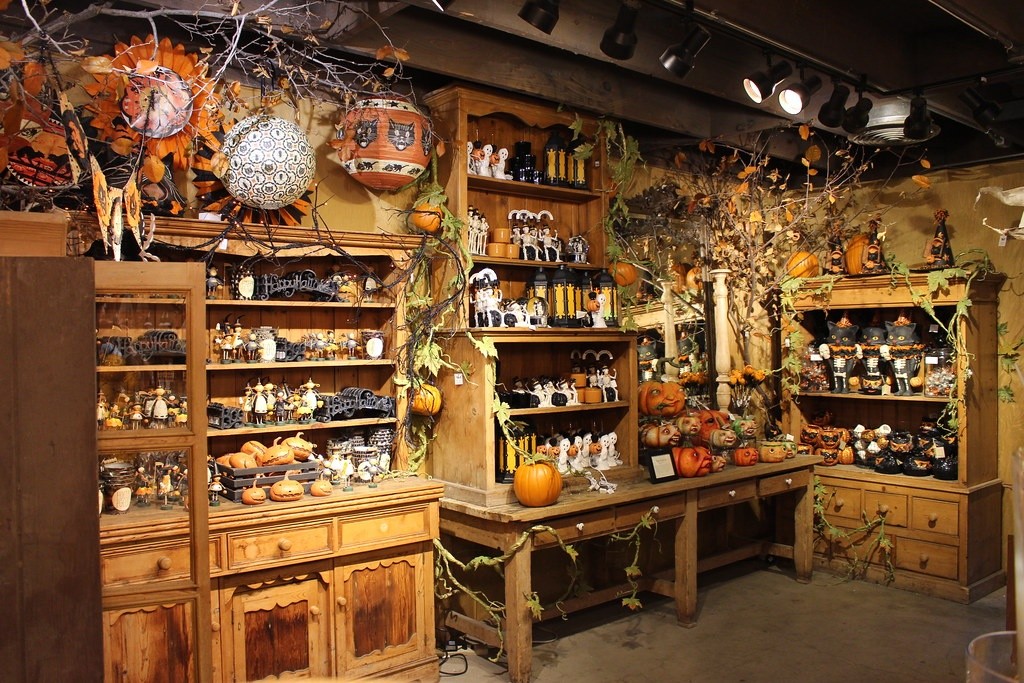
[573, 366, 619, 403]
[546, 431, 617, 474]
[470, 286, 542, 330]
[860, 218, 888, 275]
[511, 377, 581, 408]
[133, 459, 226, 507]
[927, 210, 954, 267]
[825, 217, 845, 276]
[592, 294, 607, 329]
[242, 378, 323, 424]
[576, 239, 584, 252]
[300, 331, 359, 361]
[510, 223, 563, 262]
[215, 322, 263, 359]
[467, 205, 489, 256]
[97, 386, 187, 430]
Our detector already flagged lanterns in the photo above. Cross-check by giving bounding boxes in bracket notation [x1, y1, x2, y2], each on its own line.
[217, 432, 333, 505]
[336, 88, 433, 190]
[216, 115, 316, 209]
[588, 300, 600, 311]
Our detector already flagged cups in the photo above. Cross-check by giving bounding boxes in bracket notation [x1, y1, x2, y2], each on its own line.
[493, 228, 510, 243]
[562, 372, 602, 403]
[506, 244, 520, 259]
[488, 243, 507, 258]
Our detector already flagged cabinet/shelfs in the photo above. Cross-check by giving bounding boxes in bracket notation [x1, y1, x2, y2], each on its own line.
[0, 80, 1006, 683]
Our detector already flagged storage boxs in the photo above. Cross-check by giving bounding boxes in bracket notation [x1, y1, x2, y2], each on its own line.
[212, 458, 318, 503]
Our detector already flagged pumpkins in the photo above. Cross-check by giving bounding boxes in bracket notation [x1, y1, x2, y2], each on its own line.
[411, 201, 442, 232]
[608, 260, 637, 287]
[846, 234, 871, 275]
[514, 461, 563, 507]
[408, 383, 442, 416]
[787, 251, 818, 279]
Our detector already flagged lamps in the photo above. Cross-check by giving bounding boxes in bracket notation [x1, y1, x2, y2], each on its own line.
[430, 0, 1001, 141]
